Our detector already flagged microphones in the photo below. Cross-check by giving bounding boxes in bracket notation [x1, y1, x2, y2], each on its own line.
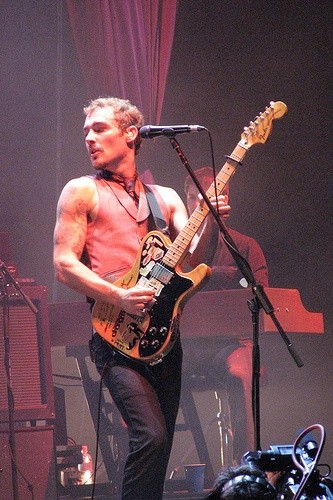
[139, 125, 204, 139]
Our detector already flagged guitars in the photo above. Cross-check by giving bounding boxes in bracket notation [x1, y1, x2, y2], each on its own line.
[87, 101, 288, 368]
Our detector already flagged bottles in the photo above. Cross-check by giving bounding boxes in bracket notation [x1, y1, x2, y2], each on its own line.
[79, 445, 93, 485]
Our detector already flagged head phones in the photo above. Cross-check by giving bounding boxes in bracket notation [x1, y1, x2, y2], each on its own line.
[205, 475, 284, 500]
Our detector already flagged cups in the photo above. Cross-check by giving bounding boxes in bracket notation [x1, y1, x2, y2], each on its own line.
[184, 464, 207, 496]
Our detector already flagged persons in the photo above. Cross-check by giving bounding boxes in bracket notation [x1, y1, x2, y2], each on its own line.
[207, 464, 281, 500]
[52, 97, 232, 500]
[107, 166, 268, 495]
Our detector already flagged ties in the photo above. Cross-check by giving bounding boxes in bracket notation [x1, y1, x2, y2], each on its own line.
[96, 168, 139, 210]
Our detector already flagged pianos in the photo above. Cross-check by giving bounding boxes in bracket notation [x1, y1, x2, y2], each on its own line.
[45, 287, 325, 348]
